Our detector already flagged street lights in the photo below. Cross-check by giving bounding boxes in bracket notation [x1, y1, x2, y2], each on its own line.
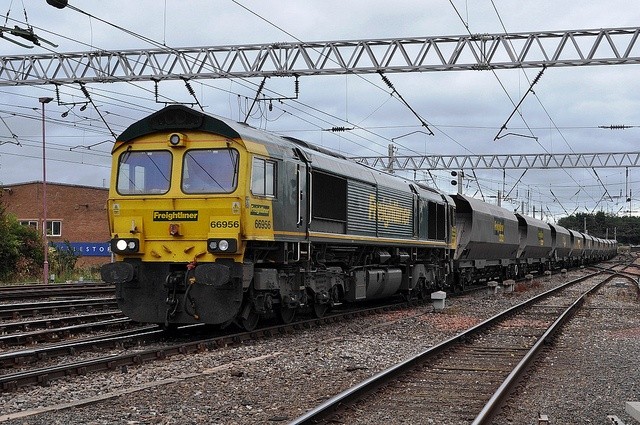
[38, 97, 54, 284]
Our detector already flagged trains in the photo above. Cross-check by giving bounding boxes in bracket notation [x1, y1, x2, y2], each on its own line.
[100, 106, 618, 331]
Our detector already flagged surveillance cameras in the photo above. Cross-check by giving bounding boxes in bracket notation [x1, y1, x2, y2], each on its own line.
[39, 97, 54, 103]
